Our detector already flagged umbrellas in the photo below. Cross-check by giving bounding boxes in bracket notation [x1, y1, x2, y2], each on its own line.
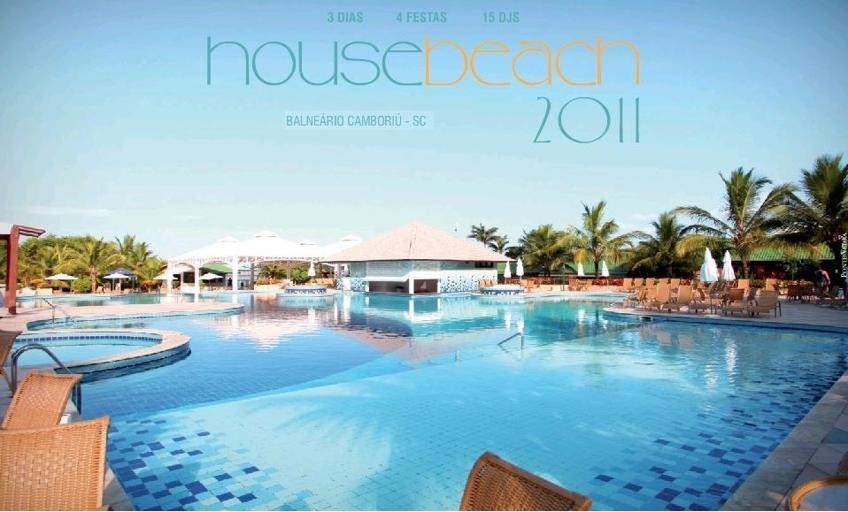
[102, 272, 131, 279]
[306, 261, 315, 285]
[601, 260, 610, 279]
[515, 257, 525, 282]
[503, 310, 511, 332]
[44, 272, 78, 282]
[577, 262, 585, 279]
[699, 246, 720, 283]
[515, 316, 524, 335]
[721, 250, 736, 283]
[198, 272, 223, 291]
[154, 273, 179, 281]
[503, 257, 511, 284]
[102, 267, 137, 275]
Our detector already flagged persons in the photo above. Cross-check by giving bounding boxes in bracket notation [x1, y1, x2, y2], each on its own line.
[814, 265, 830, 297]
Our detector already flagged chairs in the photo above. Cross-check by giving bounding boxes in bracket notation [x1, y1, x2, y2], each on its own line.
[618, 275, 816, 320]
[478, 276, 594, 292]
[0, 329, 111, 511]
[460, 449, 595, 511]
